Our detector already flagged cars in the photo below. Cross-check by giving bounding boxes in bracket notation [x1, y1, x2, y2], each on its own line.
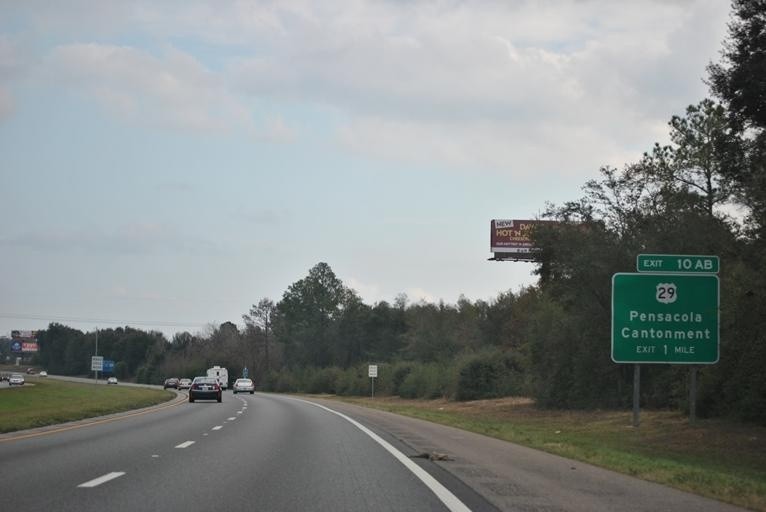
[162, 377, 256, 403]
[106, 376, 118, 384]
[0, 367, 47, 386]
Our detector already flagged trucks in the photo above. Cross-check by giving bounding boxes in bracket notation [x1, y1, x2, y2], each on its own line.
[206, 365, 229, 392]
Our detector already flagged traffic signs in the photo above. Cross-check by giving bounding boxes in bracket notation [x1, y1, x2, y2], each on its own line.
[610, 271, 722, 366]
[636, 252, 722, 275]
